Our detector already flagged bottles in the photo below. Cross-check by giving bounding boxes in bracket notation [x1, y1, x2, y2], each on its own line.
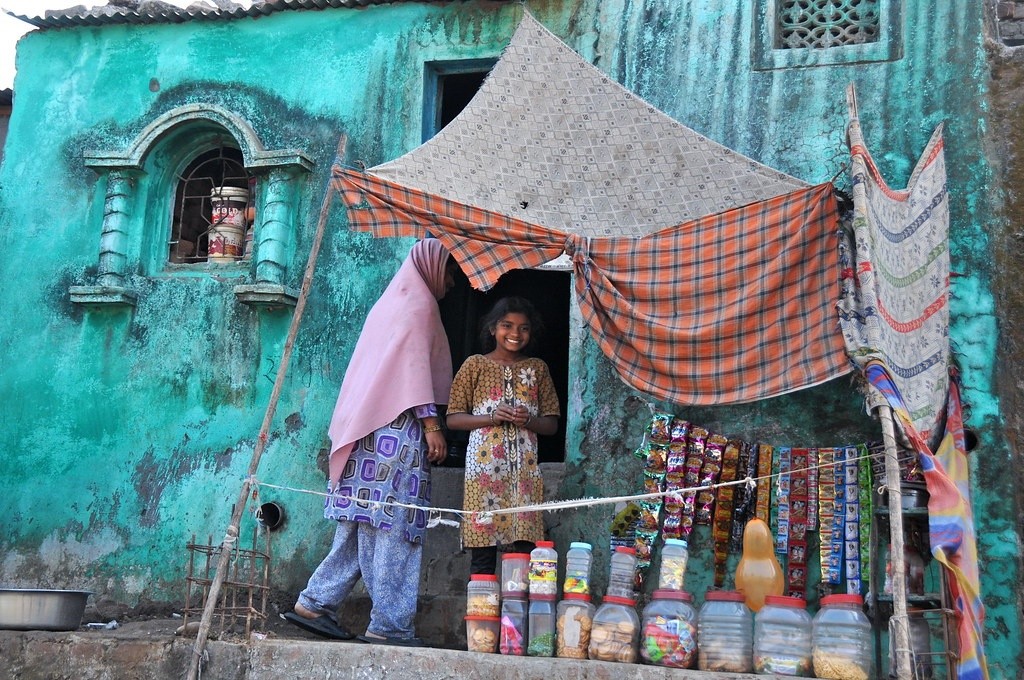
[639, 590, 699, 668]
[562, 541, 593, 594]
[464, 614, 501, 653]
[465, 574, 500, 618]
[697, 590, 754, 674]
[527, 594, 555, 658]
[887, 607, 933, 679]
[658, 538, 689, 591]
[604, 545, 637, 601]
[882, 543, 926, 596]
[502, 554, 531, 598]
[813, 594, 874, 680]
[587, 595, 640, 666]
[752, 594, 814, 676]
[498, 595, 529, 655]
[526, 541, 559, 594]
[554, 592, 597, 659]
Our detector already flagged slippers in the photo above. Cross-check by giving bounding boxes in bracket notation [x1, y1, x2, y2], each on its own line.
[285, 611, 355, 639]
[358, 635, 425, 647]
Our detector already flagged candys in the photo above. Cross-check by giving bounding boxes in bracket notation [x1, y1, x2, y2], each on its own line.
[658, 558, 685, 591]
[641, 616, 698, 669]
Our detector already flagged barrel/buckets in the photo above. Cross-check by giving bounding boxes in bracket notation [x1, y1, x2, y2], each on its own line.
[210, 186, 248, 233]
[206, 225, 245, 264]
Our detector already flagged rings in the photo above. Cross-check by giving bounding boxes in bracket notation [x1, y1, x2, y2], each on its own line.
[435, 449, 440, 453]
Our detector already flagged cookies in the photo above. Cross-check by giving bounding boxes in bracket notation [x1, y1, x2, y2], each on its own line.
[556, 614, 638, 662]
[466, 621, 496, 653]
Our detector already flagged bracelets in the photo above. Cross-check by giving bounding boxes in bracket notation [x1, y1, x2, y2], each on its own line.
[522, 411, 537, 429]
[423, 422, 442, 433]
[490, 409, 501, 427]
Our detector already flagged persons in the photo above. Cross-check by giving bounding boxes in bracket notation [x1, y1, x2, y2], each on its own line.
[285, 238, 458, 646]
[446, 297, 560, 653]
